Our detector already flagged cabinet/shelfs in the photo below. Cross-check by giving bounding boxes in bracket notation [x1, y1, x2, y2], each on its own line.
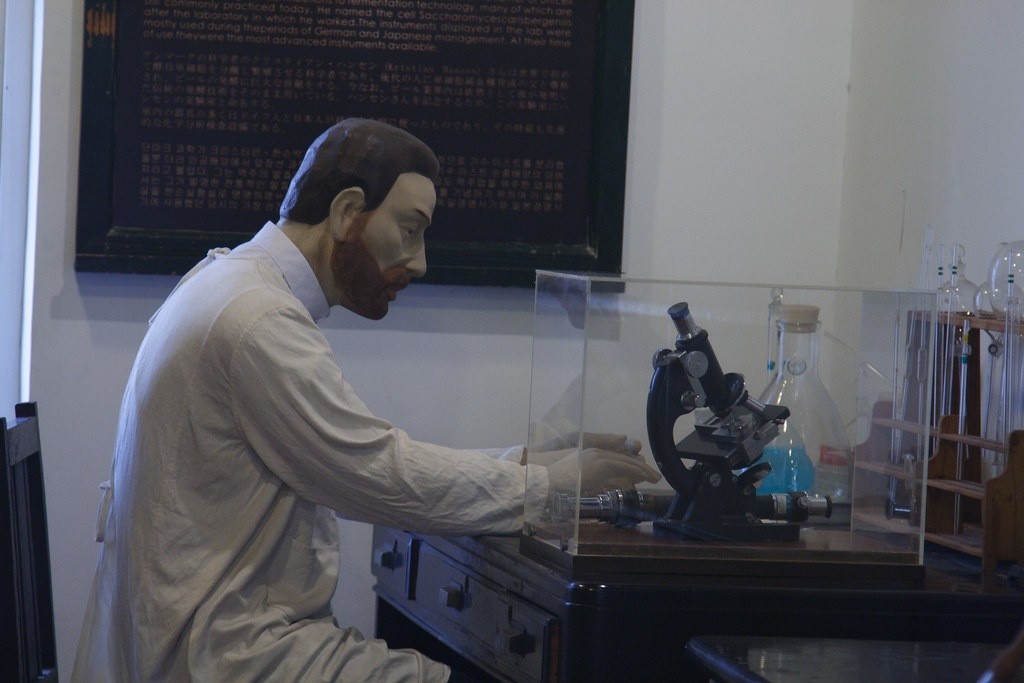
[851, 311, 1024, 579]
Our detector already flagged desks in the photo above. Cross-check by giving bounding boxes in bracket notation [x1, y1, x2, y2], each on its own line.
[371, 517, 1024, 683]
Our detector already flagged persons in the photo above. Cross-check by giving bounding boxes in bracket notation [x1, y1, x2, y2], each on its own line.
[71, 116, 666, 683]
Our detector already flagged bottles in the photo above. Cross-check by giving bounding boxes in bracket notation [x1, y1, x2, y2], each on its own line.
[756, 305, 851, 505]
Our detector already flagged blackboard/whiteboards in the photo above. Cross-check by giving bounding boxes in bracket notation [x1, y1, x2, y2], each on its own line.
[72, 0, 636, 294]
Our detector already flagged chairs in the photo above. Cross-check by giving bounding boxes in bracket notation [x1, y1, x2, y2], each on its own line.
[0, 401, 59, 683]
